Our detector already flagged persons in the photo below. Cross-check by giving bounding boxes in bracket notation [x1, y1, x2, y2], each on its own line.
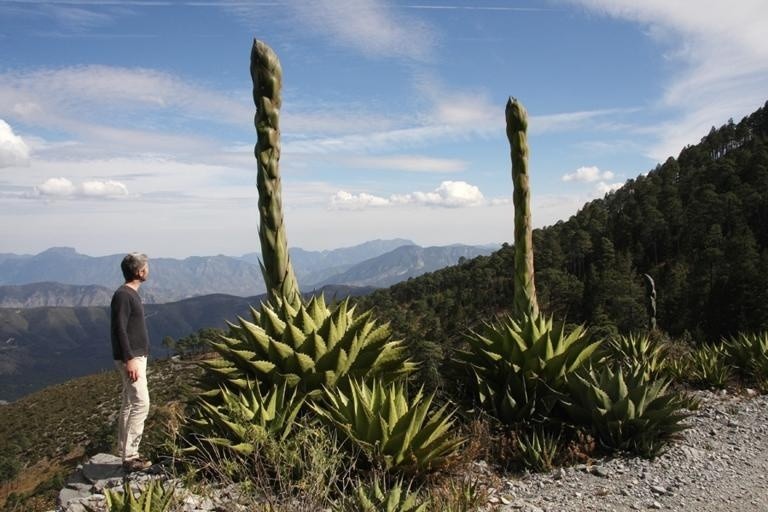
[110, 250, 153, 473]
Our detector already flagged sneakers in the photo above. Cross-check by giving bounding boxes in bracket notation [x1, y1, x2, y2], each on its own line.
[122, 457, 152, 473]
